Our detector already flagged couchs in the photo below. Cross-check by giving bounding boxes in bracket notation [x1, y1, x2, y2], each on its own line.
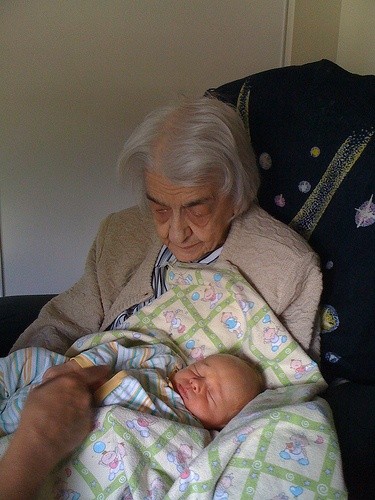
[0, 59, 375, 500]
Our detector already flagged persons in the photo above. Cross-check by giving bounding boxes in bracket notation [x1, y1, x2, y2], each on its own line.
[0, 365, 115, 500]
[7, 96, 323, 372]
[0, 340, 264, 432]
